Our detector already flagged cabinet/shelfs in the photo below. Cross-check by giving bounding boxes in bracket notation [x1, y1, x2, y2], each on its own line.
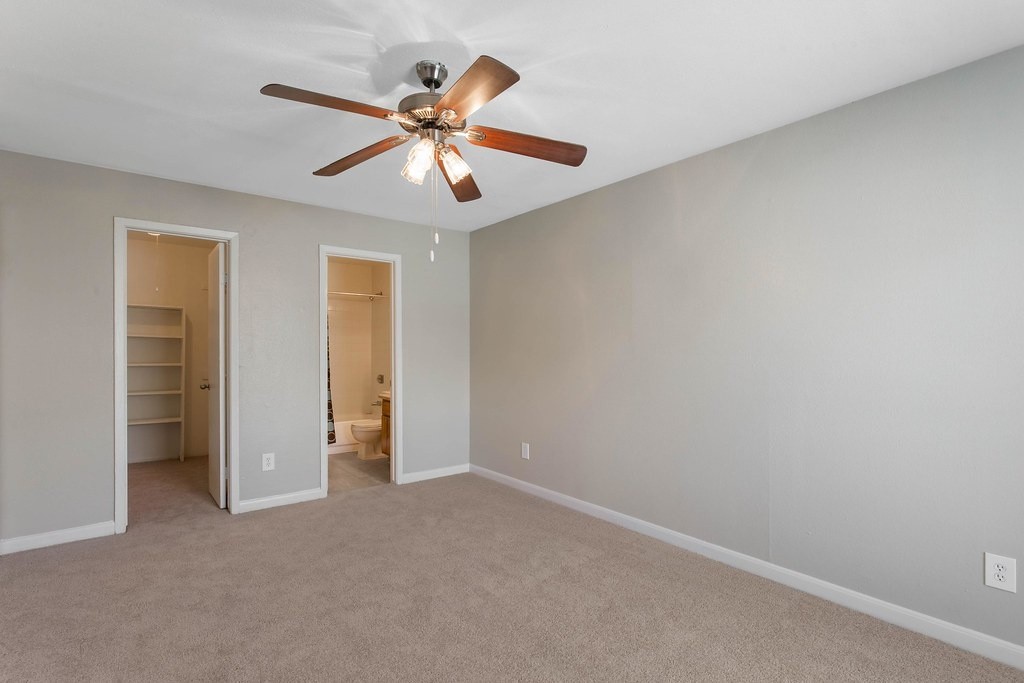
[127, 303, 186, 462]
[378, 391, 390, 456]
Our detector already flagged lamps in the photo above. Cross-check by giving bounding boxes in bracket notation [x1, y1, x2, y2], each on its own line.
[400, 129, 473, 261]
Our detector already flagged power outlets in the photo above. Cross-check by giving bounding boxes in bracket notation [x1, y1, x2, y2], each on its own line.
[984, 551, 1017, 594]
[522, 442, 530, 460]
[262, 453, 275, 472]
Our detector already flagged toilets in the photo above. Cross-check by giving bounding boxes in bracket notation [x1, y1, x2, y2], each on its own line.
[351, 419, 388, 460]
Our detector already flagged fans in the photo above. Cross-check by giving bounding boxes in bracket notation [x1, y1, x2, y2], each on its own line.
[260, 55, 588, 203]
